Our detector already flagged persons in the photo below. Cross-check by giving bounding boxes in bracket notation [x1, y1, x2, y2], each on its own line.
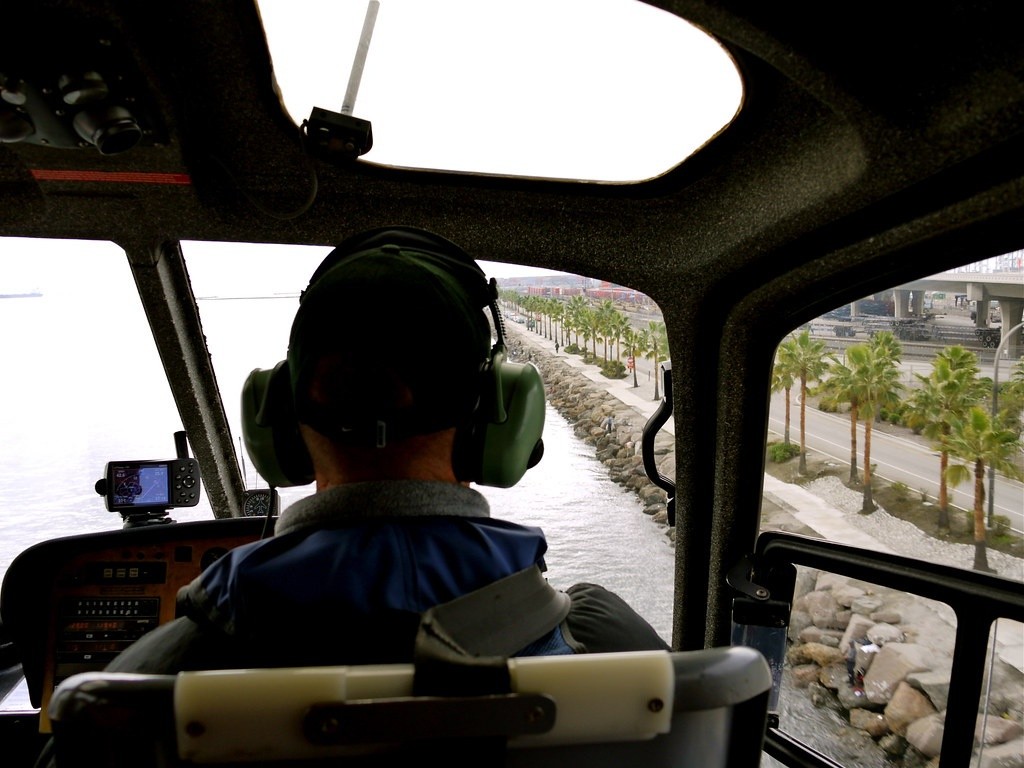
[555, 343, 559, 353]
[99, 229, 670, 673]
[846, 641, 856, 685]
[607, 416, 612, 433]
[857, 668, 865, 683]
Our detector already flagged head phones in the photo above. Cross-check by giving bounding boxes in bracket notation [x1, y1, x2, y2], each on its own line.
[242, 228, 545, 489]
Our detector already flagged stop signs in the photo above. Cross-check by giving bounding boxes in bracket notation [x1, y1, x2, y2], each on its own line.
[627, 358, 633, 363]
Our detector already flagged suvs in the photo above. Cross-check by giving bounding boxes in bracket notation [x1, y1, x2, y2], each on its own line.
[526, 319, 535, 327]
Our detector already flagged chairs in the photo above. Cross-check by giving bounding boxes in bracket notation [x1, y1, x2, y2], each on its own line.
[50, 649, 773, 765]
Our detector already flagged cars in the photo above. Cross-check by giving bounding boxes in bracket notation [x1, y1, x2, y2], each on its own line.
[503, 310, 525, 324]
